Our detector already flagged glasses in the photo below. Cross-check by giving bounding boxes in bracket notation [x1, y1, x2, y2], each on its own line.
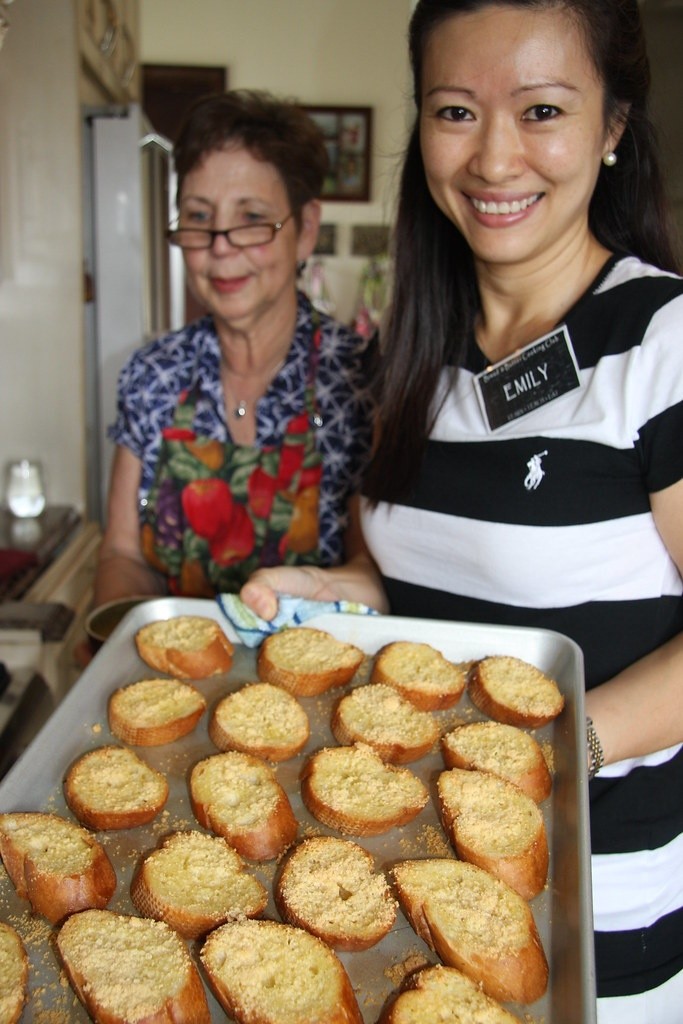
[163, 202, 306, 250]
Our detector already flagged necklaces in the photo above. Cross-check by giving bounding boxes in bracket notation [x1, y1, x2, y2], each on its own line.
[225, 355, 263, 418]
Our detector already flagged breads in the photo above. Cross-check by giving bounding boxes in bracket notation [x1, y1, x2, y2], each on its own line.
[0, 613, 564, 1024]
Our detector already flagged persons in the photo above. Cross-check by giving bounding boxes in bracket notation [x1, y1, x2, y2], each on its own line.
[75, 87, 383, 678]
[239, 2, 683, 1023]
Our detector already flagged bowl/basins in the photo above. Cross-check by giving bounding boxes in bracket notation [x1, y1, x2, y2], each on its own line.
[87, 593, 162, 653]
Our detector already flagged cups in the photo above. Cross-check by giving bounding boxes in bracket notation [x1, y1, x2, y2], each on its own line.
[7, 460, 42, 516]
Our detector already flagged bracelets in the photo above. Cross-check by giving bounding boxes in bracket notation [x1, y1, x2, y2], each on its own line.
[587, 716, 604, 779]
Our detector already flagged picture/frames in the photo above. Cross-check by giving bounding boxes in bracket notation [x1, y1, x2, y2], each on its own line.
[300, 105, 373, 201]
